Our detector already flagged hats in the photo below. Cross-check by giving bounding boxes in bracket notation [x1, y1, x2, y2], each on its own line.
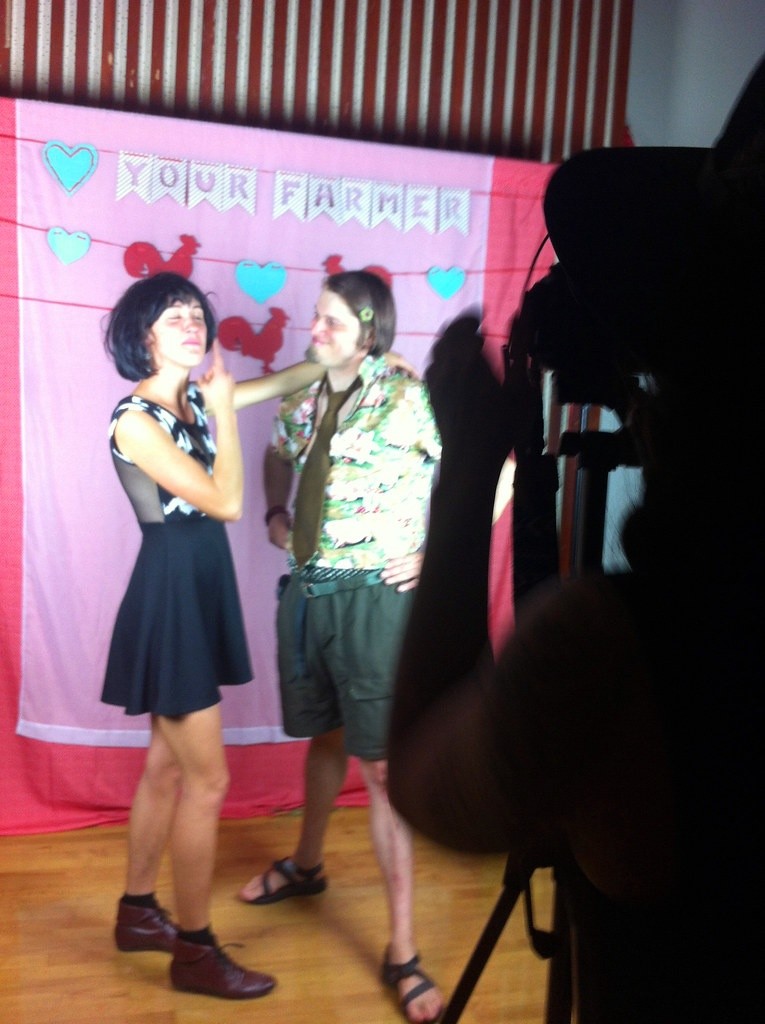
[543, 60, 765, 339]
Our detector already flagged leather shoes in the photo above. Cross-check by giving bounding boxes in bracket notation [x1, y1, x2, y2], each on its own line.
[169, 937, 277, 1000]
[115, 899, 179, 953]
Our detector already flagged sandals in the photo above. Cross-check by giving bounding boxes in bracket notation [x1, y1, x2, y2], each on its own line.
[377, 945, 443, 1024]
[250, 857, 327, 904]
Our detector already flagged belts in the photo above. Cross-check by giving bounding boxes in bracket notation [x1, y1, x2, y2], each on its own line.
[290, 571, 382, 598]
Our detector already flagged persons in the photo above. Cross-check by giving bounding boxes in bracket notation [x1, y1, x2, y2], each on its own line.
[239, 263, 520, 1024]
[101, 269, 421, 998]
[387, 53, 765, 1024]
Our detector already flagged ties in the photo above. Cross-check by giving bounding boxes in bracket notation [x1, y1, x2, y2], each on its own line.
[292, 374, 363, 568]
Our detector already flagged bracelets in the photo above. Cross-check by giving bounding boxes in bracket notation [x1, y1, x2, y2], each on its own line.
[265, 505, 290, 523]
